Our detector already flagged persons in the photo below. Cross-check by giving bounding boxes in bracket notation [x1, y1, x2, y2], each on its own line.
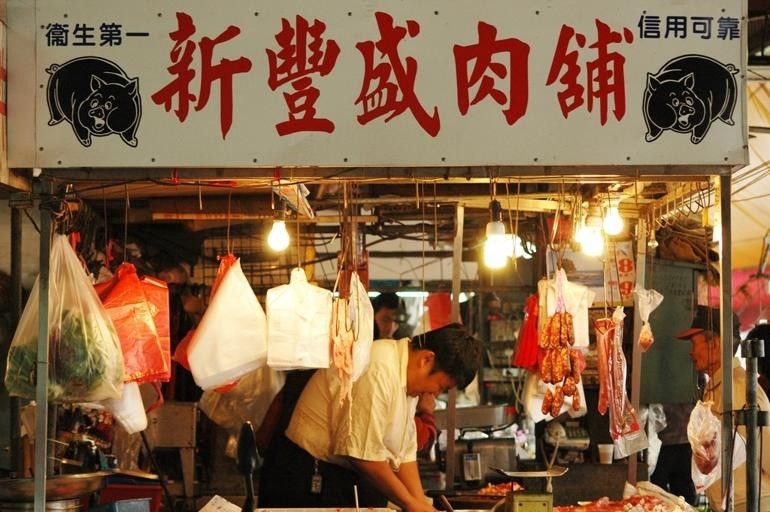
[368, 290, 411, 342]
[674, 305, 770, 512]
[252, 321, 486, 511]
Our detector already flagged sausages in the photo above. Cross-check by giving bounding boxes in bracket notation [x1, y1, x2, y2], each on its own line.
[541, 311, 581, 420]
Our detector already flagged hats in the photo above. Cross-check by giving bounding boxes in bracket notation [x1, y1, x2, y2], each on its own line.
[674, 304, 741, 340]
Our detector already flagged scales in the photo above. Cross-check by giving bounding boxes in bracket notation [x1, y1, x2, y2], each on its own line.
[433, 402, 515, 482]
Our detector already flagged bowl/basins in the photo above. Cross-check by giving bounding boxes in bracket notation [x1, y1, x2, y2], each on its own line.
[1, 470, 110, 500]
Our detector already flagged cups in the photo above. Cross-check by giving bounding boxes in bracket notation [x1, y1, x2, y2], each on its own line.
[598, 444, 615, 464]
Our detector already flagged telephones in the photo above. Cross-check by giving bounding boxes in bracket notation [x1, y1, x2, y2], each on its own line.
[544, 421, 590, 451]
